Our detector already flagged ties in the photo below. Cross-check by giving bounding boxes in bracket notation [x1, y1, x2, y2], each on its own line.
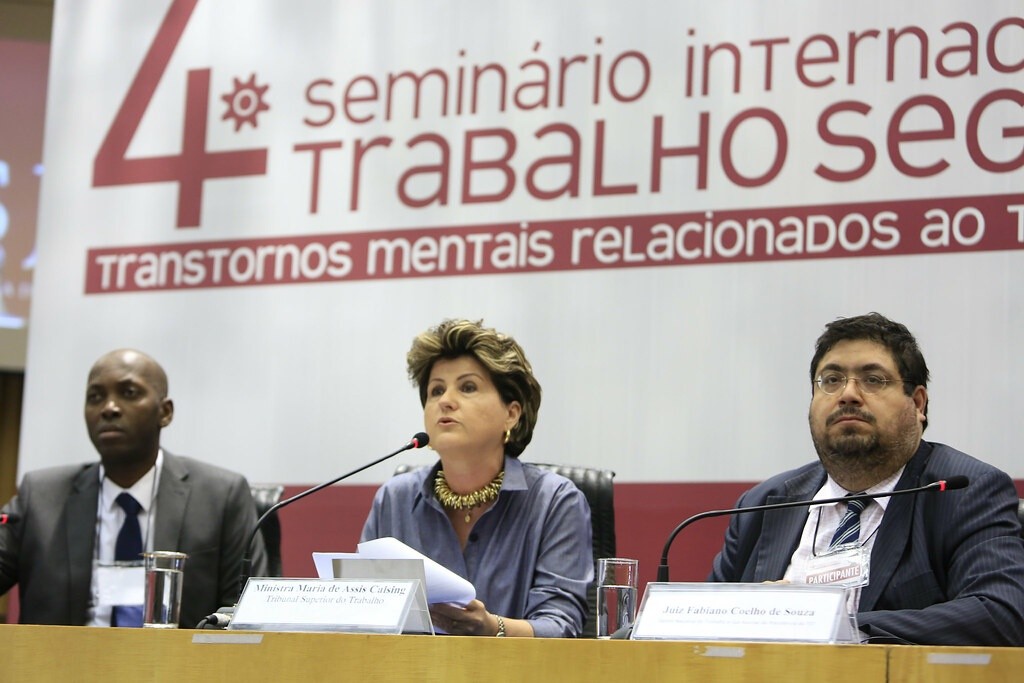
[109, 493, 146, 627]
[829, 493, 875, 546]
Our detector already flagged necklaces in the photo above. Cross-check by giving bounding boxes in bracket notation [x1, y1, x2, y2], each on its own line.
[434, 469, 505, 524]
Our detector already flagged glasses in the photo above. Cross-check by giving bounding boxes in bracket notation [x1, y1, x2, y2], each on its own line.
[812, 371, 915, 396]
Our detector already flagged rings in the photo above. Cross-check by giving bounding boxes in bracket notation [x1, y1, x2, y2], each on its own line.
[452, 621, 458, 627]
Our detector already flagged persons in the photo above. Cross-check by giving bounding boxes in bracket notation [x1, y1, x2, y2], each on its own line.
[0, 348, 269, 630]
[355, 319, 595, 639]
[707, 311, 1024, 647]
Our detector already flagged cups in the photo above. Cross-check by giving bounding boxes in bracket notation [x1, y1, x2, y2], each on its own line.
[596, 557, 639, 639]
[143, 551, 187, 628]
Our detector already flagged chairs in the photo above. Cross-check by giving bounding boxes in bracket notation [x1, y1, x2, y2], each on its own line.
[522, 463, 616, 639]
[251, 486, 283, 577]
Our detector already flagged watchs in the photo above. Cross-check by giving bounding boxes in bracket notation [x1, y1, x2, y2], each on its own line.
[495, 614, 507, 637]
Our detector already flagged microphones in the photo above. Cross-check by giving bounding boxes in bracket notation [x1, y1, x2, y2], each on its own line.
[657, 476, 970, 582]
[0, 513, 20, 525]
[239, 432, 430, 593]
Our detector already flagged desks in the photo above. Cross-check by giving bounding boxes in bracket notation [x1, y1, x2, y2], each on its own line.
[0, 624, 1024, 683]
